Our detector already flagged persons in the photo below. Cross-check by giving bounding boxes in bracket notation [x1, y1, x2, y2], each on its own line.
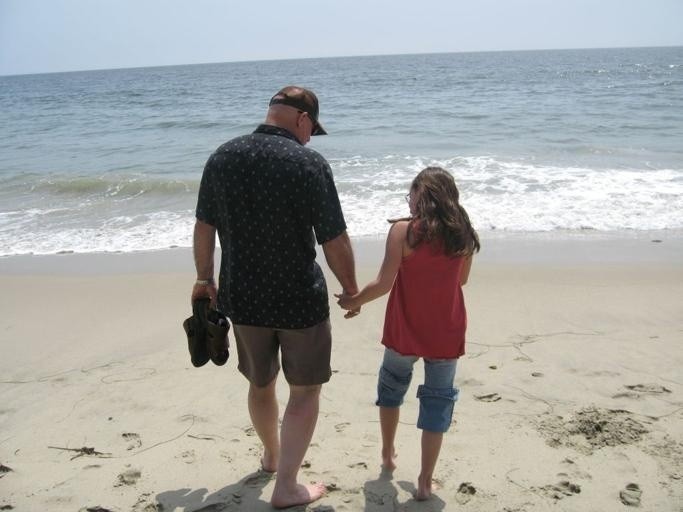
[193, 88, 361, 507]
[332, 164, 479, 500]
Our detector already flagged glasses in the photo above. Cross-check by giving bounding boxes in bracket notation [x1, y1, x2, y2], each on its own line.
[298, 110, 319, 136]
[405, 191, 412, 203]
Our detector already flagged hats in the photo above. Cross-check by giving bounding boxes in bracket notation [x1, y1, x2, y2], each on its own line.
[267, 84, 328, 136]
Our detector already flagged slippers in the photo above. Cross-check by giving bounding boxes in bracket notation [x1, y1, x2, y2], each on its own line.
[182, 296, 231, 368]
